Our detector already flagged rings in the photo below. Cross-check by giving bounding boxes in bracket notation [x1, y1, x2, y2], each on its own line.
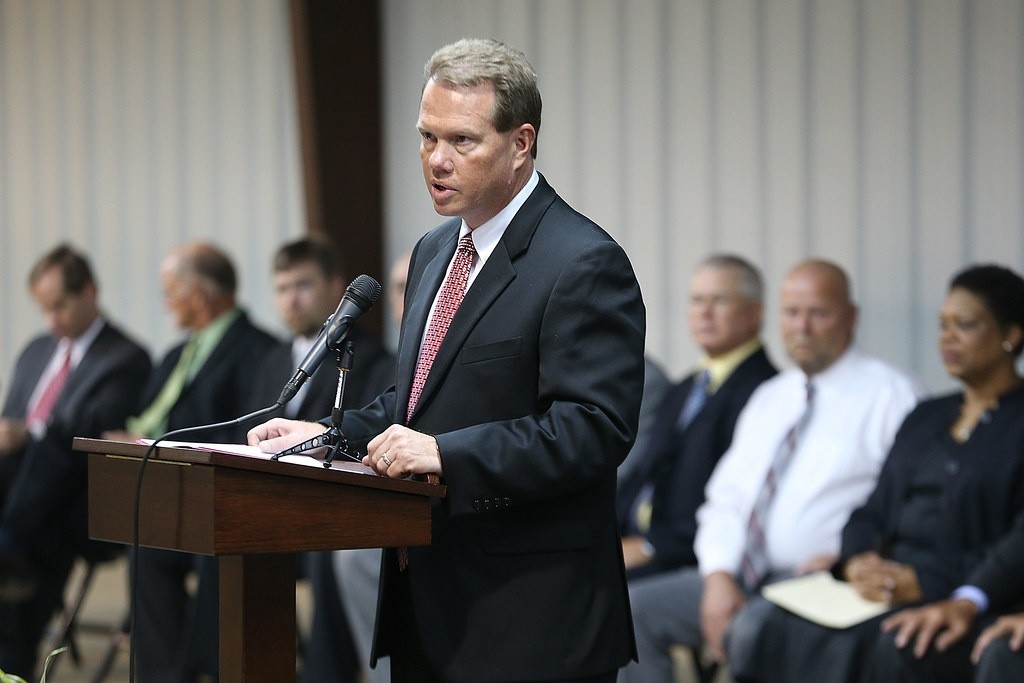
[383, 452, 391, 465]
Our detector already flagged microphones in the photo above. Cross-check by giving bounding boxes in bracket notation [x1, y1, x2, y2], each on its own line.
[277, 274, 382, 407]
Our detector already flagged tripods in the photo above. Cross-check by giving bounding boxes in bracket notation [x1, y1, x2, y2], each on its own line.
[271, 339, 366, 468]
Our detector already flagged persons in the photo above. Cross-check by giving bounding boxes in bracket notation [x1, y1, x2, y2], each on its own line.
[619, 252, 1024, 683]
[0, 239, 396, 683]
[248, 42, 643, 683]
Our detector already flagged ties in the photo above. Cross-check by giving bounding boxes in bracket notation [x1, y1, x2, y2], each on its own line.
[31, 340, 75, 424]
[395, 231, 477, 573]
[625, 370, 709, 536]
[125, 337, 198, 440]
[741, 379, 816, 591]
